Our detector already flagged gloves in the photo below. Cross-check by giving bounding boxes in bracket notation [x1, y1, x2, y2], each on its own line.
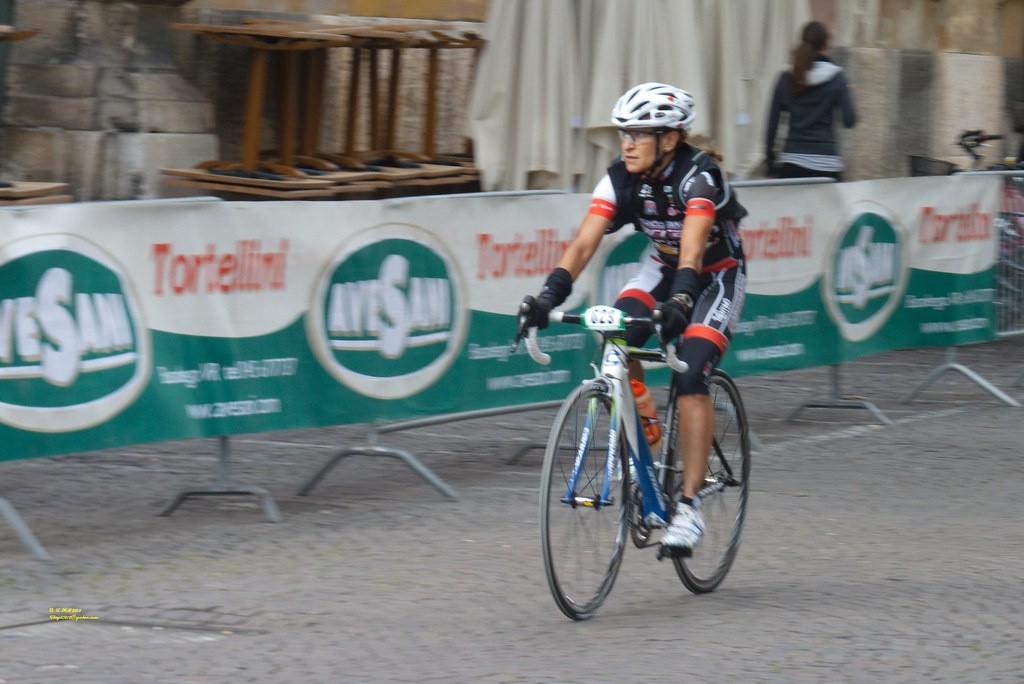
[648, 297, 690, 345]
[518, 295, 551, 330]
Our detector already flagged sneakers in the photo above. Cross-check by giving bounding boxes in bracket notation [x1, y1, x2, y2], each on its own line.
[662, 504, 706, 549]
[617, 458, 635, 481]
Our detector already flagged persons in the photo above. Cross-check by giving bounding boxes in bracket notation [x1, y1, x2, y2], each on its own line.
[764, 21, 857, 178]
[515, 81, 750, 544]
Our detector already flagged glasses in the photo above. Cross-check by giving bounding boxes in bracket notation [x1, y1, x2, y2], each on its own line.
[617, 129, 660, 144]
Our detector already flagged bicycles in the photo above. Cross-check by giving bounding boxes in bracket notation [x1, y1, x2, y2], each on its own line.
[957, 126, 1024, 328]
[512, 312, 754, 624]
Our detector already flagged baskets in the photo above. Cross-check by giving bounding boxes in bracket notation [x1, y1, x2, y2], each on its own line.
[909, 154, 955, 176]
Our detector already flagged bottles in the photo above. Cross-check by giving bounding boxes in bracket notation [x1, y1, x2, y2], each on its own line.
[631, 379, 663, 449]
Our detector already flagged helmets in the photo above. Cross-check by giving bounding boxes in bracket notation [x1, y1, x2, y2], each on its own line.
[611, 82, 695, 133]
